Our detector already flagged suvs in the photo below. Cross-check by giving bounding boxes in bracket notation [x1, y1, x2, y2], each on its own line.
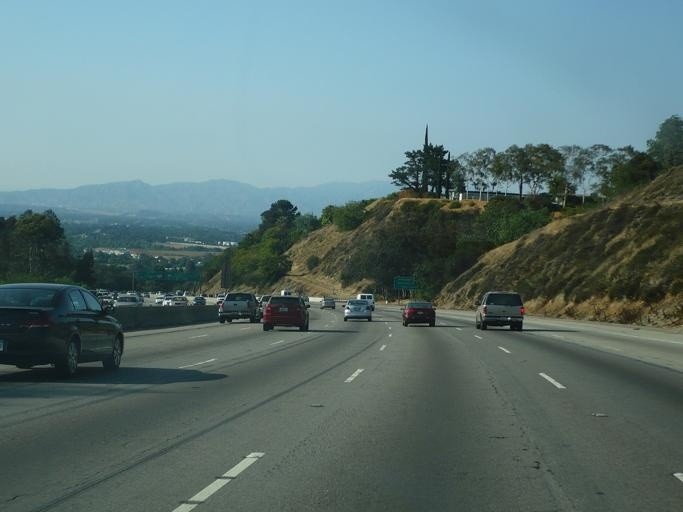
[474, 291, 525, 332]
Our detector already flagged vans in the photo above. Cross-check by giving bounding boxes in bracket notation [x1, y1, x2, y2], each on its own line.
[357, 294, 375, 310]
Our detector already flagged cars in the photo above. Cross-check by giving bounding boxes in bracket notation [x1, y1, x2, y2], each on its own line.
[342, 299, 372, 321]
[0, 282, 123, 375]
[400, 301, 435, 328]
[319, 296, 335, 310]
[91, 287, 311, 332]
